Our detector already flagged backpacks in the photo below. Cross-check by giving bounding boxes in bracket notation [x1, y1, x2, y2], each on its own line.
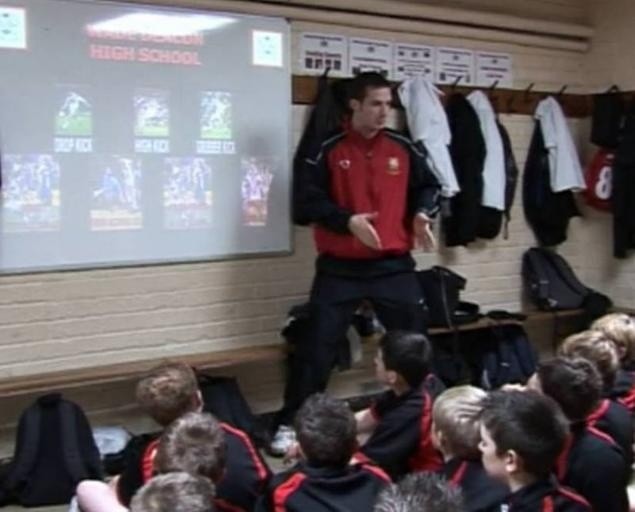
[519, 244, 593, 310]
[479, 322, 539, 389]
[0, 391, 105, 508]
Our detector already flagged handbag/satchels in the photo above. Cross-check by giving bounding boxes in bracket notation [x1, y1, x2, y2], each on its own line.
[417, 265, 481, 330]
[195, 369, 255, 438]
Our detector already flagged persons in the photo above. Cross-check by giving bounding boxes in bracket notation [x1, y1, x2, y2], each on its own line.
[268, 70, 443, 458]
[0, 310, 635, 512]
